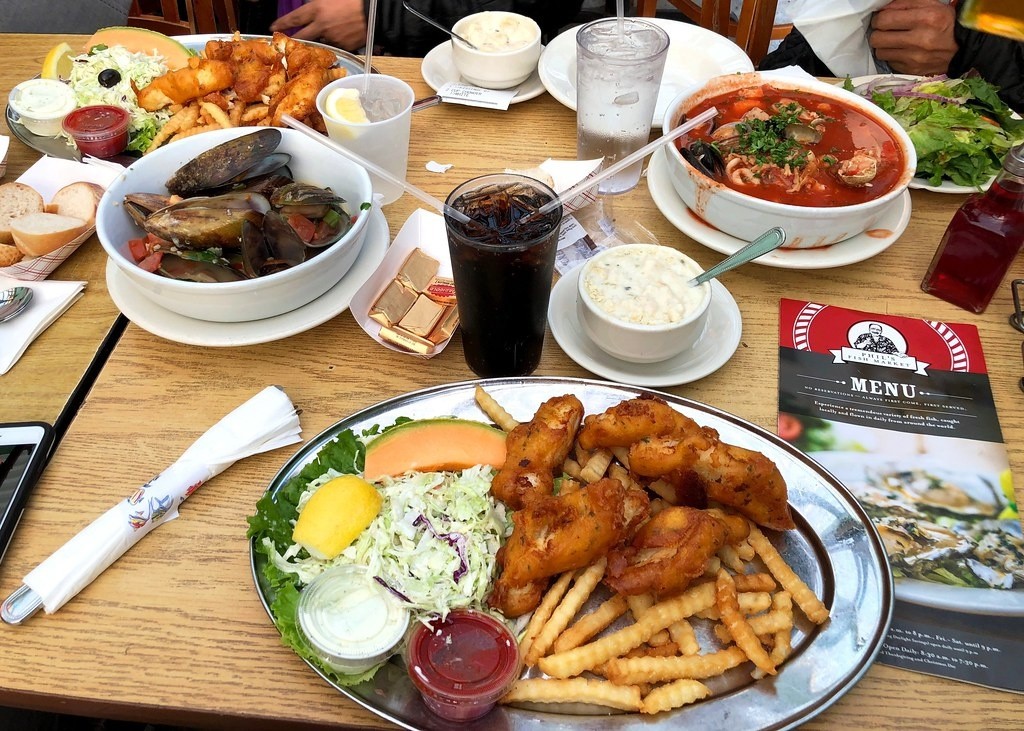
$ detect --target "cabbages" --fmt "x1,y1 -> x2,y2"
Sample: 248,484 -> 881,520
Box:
65,45 -> 173,130
266,466 -> 513,633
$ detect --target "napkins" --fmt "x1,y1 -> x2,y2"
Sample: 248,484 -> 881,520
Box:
23,386 -> 303,615
0,276 -> 89,377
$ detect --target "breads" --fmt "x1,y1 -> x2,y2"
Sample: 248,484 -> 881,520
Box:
0,182 -> 106,267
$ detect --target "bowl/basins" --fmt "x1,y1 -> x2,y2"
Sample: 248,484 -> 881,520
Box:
95,125 -> 373,324
576,242 -> 713,364
405,602 -> 522,723
293,560 -> 412,677
62,105 -> 131,159
662,71 -> 917,249
451,11 -> 541,90
9,78 -> 77,138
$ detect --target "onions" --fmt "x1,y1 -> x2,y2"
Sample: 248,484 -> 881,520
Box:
853,74 -> 959,104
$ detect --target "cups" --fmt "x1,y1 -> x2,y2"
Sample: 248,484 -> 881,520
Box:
576,18 -> 670,196
315,74 -> 416,207
442,173 -> 564,380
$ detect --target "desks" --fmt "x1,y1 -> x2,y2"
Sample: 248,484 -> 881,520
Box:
0,31 -> 122,428
0,51 -> 1024,731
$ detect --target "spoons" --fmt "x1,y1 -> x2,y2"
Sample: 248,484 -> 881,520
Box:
0,287 -> 34,324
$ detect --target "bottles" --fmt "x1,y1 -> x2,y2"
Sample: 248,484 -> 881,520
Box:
920,141 -> 1024,317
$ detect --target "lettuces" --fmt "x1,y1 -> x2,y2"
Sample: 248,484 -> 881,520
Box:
89,43 -> 158,149
875,67 -> 1024,187
244,416 -> 414,687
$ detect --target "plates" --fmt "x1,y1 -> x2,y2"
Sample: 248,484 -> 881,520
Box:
646,145 -> 913,270
536,17 -> 755,129
105,200 -> 389,348
830,73 -> 1022,194
250,377 -> 898,731
5,34 -> 382,168
547,260 -> 743,387
421,40 -> 547,104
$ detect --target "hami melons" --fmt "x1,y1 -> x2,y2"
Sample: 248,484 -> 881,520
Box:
364,419 -> 509,481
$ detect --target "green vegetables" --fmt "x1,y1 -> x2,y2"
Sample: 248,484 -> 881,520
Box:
708,101 -> 839,178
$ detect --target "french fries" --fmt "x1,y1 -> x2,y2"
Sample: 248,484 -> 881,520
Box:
474,384 -> 831,712
143,98 -> 271,155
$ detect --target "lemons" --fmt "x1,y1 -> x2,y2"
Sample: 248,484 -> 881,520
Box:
41,42 -> 74,82
291,474 -> 383,560
325,87 -> 372,127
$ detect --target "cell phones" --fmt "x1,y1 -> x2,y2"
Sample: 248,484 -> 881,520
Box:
0,420 -> 56,563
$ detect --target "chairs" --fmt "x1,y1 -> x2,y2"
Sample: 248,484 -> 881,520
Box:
625,0 -> 794,71
126,0 -> 237,36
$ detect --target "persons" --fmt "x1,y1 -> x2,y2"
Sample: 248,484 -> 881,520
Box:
270,0 -> 583,59
758,0 -> 1024,115
